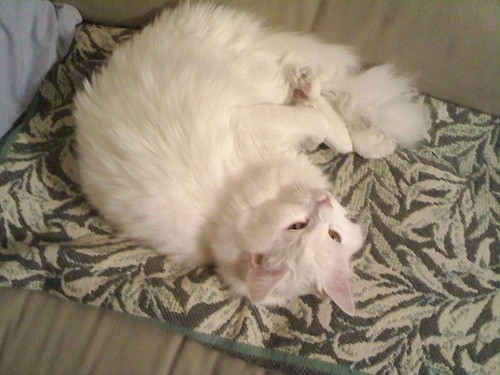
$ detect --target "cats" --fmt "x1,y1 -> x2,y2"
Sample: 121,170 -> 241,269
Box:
70,0 -> 431,317
